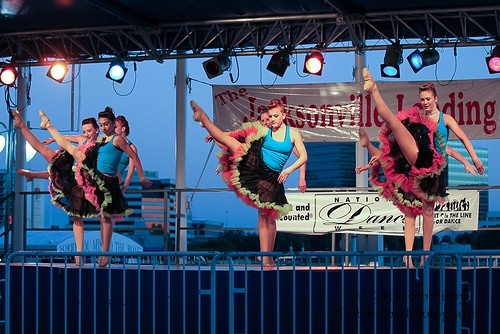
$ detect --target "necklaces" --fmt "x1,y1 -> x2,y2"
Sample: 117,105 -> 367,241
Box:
427,112 -> 438,116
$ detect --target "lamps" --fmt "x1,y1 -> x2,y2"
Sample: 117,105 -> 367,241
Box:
202,54 -> 232,80
303,52 -> 324,76
266,50 -> 291,77
105,58 -> 128,84
0,65 -> 17,87
407,40 -> 440,73
380,41 -> 404,78
485,45 -> 500,74
46,61 -> 69,83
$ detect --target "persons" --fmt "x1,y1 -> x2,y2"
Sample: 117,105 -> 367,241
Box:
12,108 -> 154,267
356,127 -> 417,268
189,98 -> 310,267
362,67 -> 484,267
